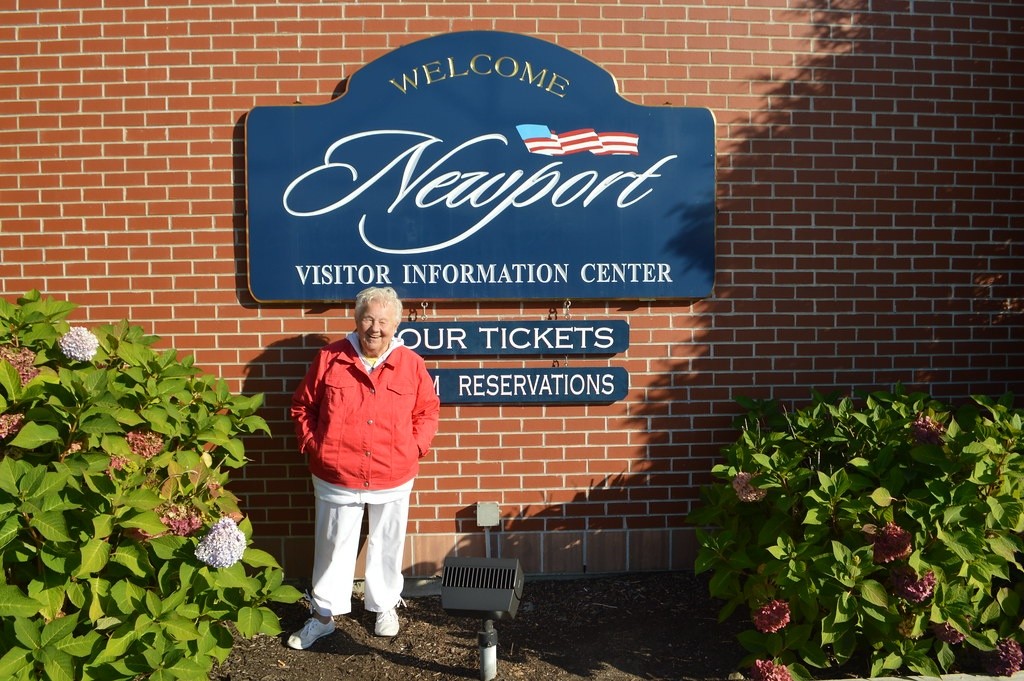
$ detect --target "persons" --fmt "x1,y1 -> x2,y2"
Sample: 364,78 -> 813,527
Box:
287,287 -> 440,649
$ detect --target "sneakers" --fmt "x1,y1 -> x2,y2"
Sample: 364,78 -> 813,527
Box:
375,608 -> 400,637
287,616 -> 335,650
